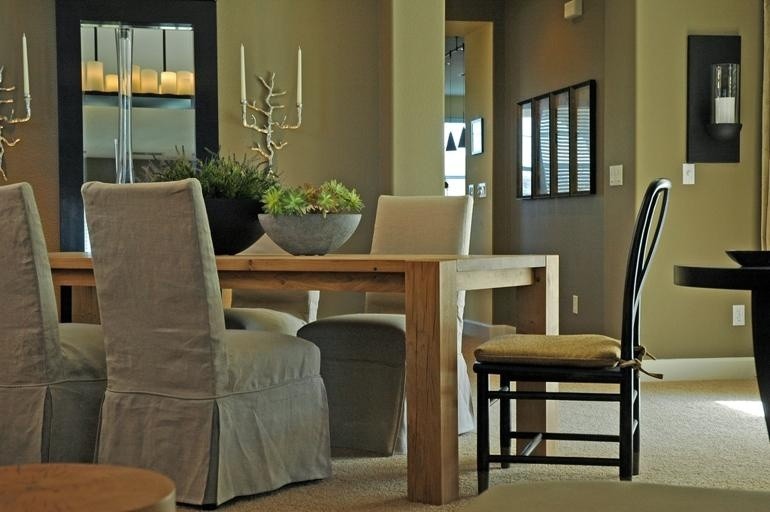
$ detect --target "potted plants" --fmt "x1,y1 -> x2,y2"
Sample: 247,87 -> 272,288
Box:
256,180 -> 364,255
137,152 -> 282,255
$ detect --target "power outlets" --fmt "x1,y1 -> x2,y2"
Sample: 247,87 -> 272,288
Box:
609,164 -> 623,186
572,294 -> 578,314
733,304 -> 745,326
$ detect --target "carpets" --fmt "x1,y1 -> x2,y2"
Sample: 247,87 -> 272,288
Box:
466,480 -> 769,512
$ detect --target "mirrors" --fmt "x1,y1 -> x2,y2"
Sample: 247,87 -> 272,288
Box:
514,79 -> 596,200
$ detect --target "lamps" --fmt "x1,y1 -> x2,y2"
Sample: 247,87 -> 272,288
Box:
457,44 -> 467,149
81,22 -> 196,97
445,51 -> 457,152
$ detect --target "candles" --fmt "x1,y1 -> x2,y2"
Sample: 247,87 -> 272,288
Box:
20,34 -> 29,97
295,45 -> 304,107
240,41 -> 246,101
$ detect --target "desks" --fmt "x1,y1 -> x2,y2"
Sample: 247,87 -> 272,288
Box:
674,265 -> 770,438
46,251 -> 560,506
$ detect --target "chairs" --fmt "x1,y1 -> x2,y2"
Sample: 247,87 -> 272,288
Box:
0,182 -> 110,465
81,180 -> 328,501
299,195 -> 474,453
472,179 -> 672,488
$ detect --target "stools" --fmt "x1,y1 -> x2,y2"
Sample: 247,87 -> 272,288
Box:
0,463 -> 178,511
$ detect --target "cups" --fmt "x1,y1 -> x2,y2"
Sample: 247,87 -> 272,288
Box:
80,61 -> 194,95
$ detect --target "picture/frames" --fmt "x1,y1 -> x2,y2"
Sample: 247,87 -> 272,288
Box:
470,117 -> 484,156
